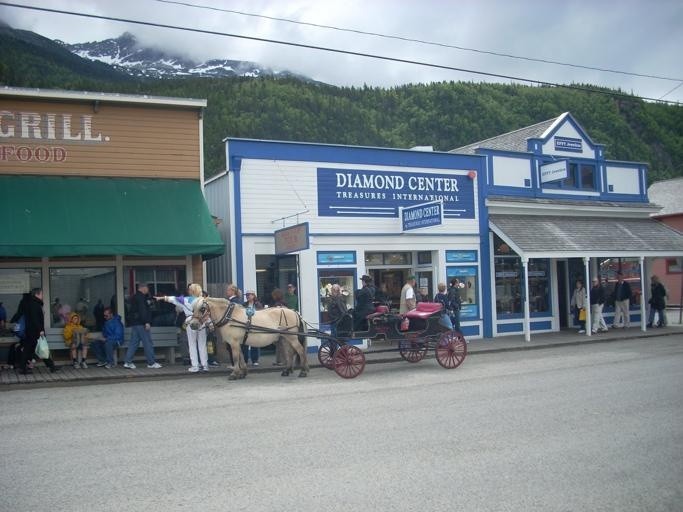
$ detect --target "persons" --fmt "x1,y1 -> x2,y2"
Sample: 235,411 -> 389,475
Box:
2,274 -> 460,374
590,278 -> 608,333
646,275 -> 667,328
571,278 -> 587,334
612,273 -> 633,330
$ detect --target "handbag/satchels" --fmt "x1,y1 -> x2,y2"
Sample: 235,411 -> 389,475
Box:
73,328 -> 87,350
207,333 -> 217,355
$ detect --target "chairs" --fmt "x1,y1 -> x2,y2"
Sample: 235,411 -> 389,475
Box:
364,306 -> 389,320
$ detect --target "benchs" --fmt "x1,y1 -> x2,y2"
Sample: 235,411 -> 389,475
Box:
406,302 -> 443,328
45,327 -> 178,364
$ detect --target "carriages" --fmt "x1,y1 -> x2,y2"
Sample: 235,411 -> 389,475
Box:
189,295 -> 467,378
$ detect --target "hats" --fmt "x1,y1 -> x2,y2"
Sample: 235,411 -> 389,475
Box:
244,289 -> 255,294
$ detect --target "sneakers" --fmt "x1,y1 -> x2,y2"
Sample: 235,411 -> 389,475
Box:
96,361 -> 112,368
20,368 -> 33,374
148,363 -> 162,368
210,361 -> 219,367
51,366 -> 61,373
189,363 -> 209,372
73,361 -> 88,369
123,363 -> 136,369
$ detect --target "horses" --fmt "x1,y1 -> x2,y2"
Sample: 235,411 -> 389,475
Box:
190,292 -> 310,380
